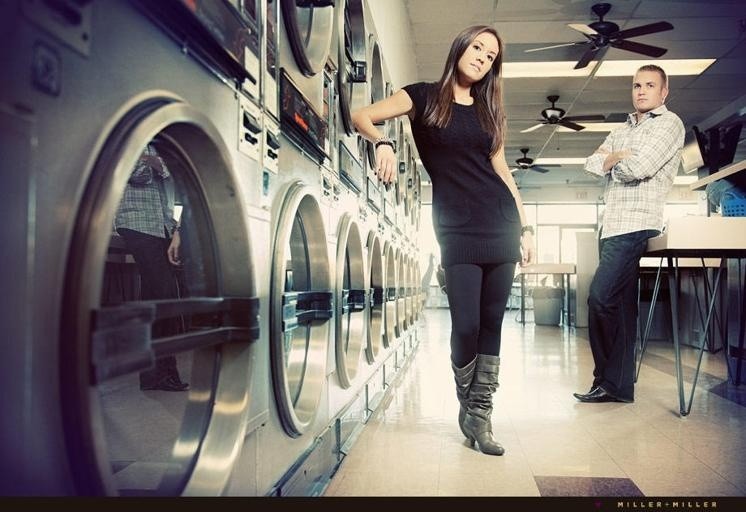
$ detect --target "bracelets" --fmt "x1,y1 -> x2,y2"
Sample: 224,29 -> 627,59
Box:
170,225 -> 183,234
376,136 -> 396,152
521,225 -> 533,235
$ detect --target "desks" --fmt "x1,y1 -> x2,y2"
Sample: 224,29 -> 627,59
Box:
514,261 -> 575,327
635,256 -> 722,354
634,216 -> 744,416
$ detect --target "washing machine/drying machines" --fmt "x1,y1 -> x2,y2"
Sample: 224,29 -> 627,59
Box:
265,0 -> 343,494
0,0 -> 264,512
368,4 -> 421,426
340,1 -> 371,455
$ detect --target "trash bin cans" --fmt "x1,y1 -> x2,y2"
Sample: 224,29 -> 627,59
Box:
532,286 -> 564,325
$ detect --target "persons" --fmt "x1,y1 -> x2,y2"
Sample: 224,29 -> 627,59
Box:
168,208 -> 215,332
349,25 -> 533,454
573,65 -> 685,402
115,143 -> 189,391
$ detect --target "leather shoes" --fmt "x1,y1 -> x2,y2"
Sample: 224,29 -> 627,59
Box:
574,387 -> 625,403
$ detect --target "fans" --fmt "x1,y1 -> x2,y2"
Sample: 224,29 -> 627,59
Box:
519,96 -> 605,133
524,3 -> 675,70
509,149 -> 561,173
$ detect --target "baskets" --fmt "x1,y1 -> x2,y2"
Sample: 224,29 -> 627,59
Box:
721,192 -> 746,217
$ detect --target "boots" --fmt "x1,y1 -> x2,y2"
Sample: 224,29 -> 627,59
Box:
463,354 -> 505,455
450,353 -> 478,440
